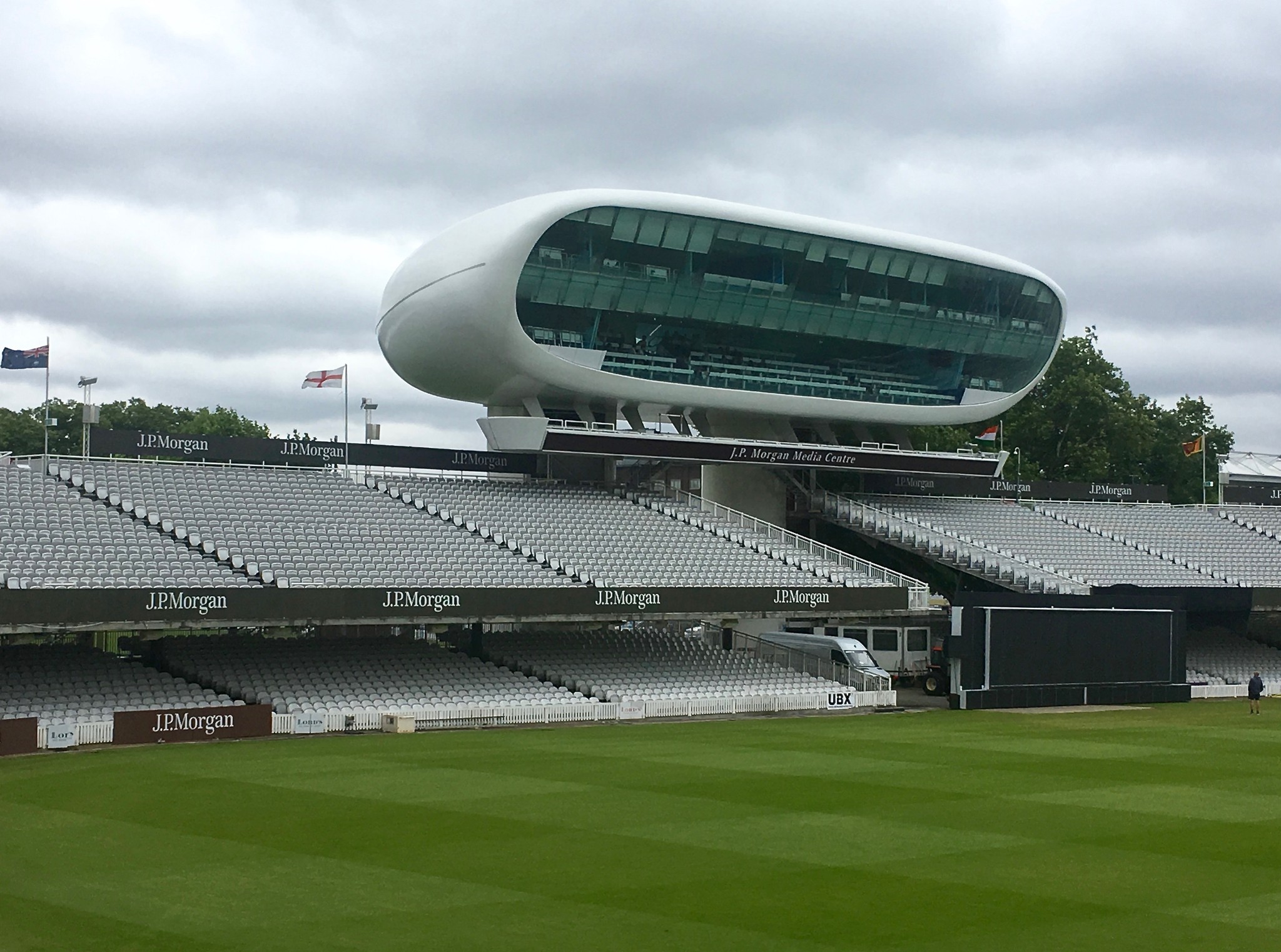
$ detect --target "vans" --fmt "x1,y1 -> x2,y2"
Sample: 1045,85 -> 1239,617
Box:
754,631 -> 890,691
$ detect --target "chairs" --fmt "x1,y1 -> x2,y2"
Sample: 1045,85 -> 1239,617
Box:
1186,639 -> 1281,685
0,628 -> 855,726
811,493 -> 1281,595
0,464 -> 897,589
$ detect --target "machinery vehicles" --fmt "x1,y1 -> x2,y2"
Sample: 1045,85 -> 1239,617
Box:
921,646 -> 951,697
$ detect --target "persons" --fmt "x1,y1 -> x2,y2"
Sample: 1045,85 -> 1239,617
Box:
1248,672 -> 1263,714
597,322 -> 768,384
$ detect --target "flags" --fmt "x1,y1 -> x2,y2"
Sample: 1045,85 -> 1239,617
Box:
0,346 -> 50,369
975,425 -> 998,441
301,366 -> 346,389
1182,436 -> 1203,457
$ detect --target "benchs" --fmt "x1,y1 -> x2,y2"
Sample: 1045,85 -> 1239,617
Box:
596,341 -> 956,407
